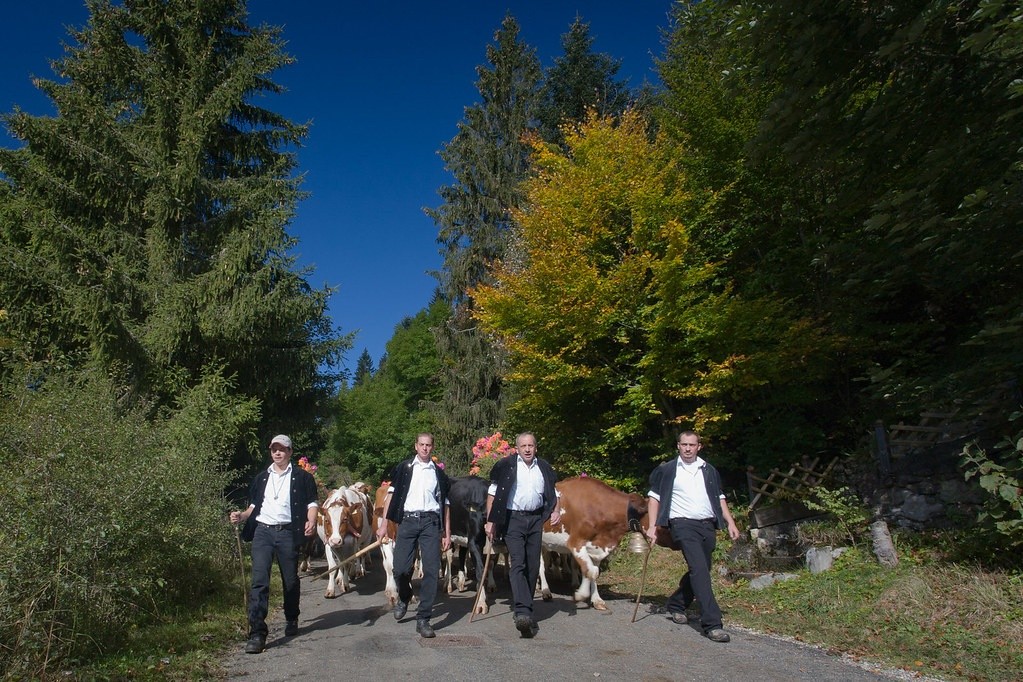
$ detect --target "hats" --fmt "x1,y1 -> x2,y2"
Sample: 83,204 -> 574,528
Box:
268,435 -> 292,449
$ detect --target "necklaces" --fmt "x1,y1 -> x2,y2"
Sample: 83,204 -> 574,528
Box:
272,471 -> 287,499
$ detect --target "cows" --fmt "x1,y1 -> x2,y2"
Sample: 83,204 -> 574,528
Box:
550,551 -> 580,588
486,544 -> 510,593
414,550 -> 424,579
536,476 -> 681,610
439,475 -> 498,614
373,485 -> 417,605
316,482 -> 375,599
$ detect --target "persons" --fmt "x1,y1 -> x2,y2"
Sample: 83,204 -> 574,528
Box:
485,432 -> 561,638
647,432 -> 739,642
376,433 -> 451,638
230,435 -> 318,653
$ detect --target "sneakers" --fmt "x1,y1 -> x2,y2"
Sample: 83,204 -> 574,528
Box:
245,636 -> 264,653
393,599 -> 407,620
416,619 -> 435,638
515,615 -> 535,638
707,628 -> 730,641
285,617 -> 299,637
672,611 -> 688,624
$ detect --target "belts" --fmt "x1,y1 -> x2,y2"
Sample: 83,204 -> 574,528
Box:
258,522 -> 292,531
402,511 -> 436,517
507,507 -> 541,515
